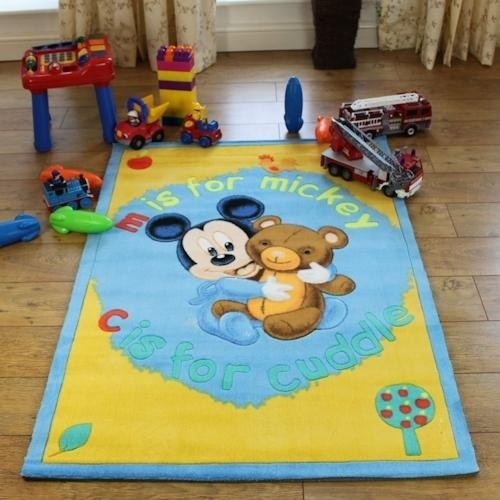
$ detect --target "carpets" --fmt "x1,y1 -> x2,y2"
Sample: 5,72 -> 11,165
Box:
22,139 -> 481,480
